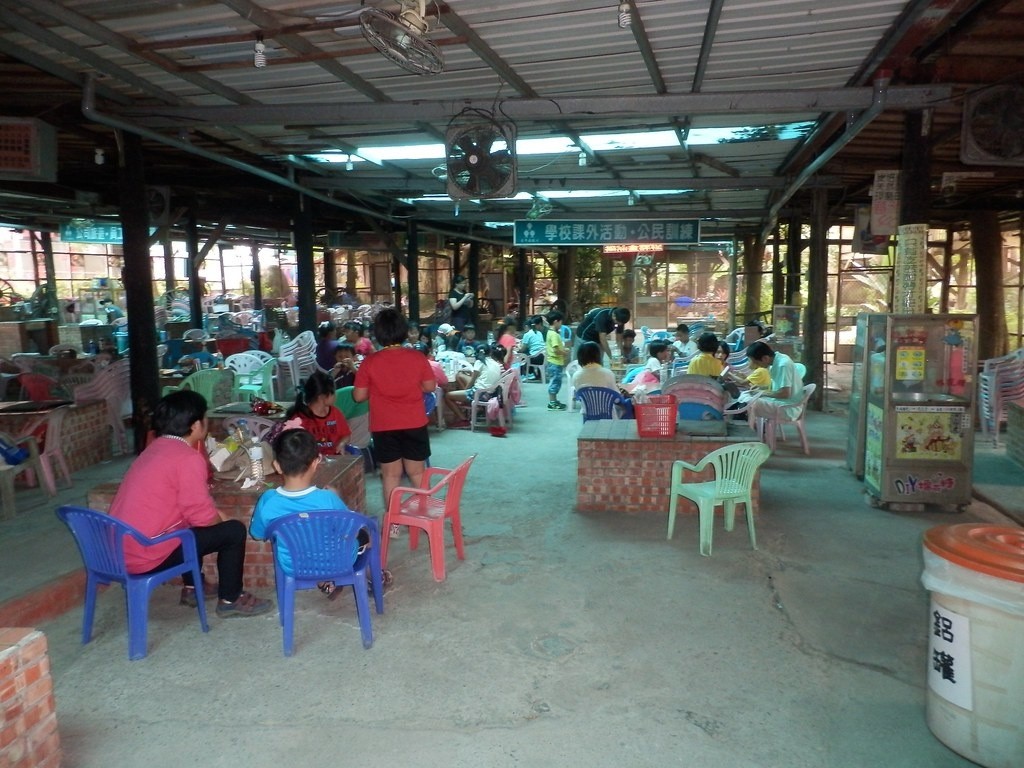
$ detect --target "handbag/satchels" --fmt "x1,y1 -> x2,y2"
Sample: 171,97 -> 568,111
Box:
213,438 -> 277,481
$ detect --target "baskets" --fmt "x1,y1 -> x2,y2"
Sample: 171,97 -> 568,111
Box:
634,394 -> 676,438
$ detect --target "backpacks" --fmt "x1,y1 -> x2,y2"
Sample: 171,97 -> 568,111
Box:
510,367 -> 523,404
0,432 -> 30,466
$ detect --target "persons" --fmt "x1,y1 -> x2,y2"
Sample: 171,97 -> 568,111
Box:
105,391 -> 272,618
315,274 -> 805,432
248,428 -> 393,601
100,298 -> 123,320
353,307 -> 435,540
286,371 -> 352,456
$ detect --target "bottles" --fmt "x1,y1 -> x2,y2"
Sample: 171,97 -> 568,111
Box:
248,437 -> 263,480
88,338 -> 96,354
438,355 -> 459,375
98,338 -> 105,351
236,419 -> 250,441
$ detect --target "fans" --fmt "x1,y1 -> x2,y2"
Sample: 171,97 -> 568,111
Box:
961,85 -> 1024,167
446,122 -> 519,201
146,185 -> 170,227
359,0 -> 445,76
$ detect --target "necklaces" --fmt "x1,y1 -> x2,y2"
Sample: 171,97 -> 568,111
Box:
384,344 -> 401,349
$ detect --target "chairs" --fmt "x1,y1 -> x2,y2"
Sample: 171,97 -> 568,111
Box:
977,347 -> 1024,449
0,304 -> 816,662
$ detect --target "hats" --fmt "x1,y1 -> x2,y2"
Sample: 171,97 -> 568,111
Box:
99,297 -> 114,305
437,324 -> 460,337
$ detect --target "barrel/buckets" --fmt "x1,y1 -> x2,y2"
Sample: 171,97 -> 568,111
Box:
920,523 -> 1024,768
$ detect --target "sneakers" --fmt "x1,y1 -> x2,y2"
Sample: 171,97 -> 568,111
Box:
216,590 -> 274,618
179,573 -> 219,609
547,401 -> 567,411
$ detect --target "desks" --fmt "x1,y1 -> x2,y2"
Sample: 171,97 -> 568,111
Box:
1,399 -> 112,484
0,626 -> 62,768
611,364 -> 640,383
0,319 -> 59,359
619,382 -> 663,394
206,402 -> 297,442
37,355 -> 95,374
165,322 -> 189,339
58,325 -> 114,352
430,377 -> 468,424
86,454 -> 368,588
577,419 -> 761,517
474,320 -> 498,340
159,368 -> 233,408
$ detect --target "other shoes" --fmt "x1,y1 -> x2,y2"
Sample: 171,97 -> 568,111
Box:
521,374 -> 527,382
527,371 -> 535,379
368,568 -> 393,597
318,581 -> 343,602
513,399 -> 528,408
388,523 -> 400,539
447,420 -> 470,429
436,419 -> 446,431
